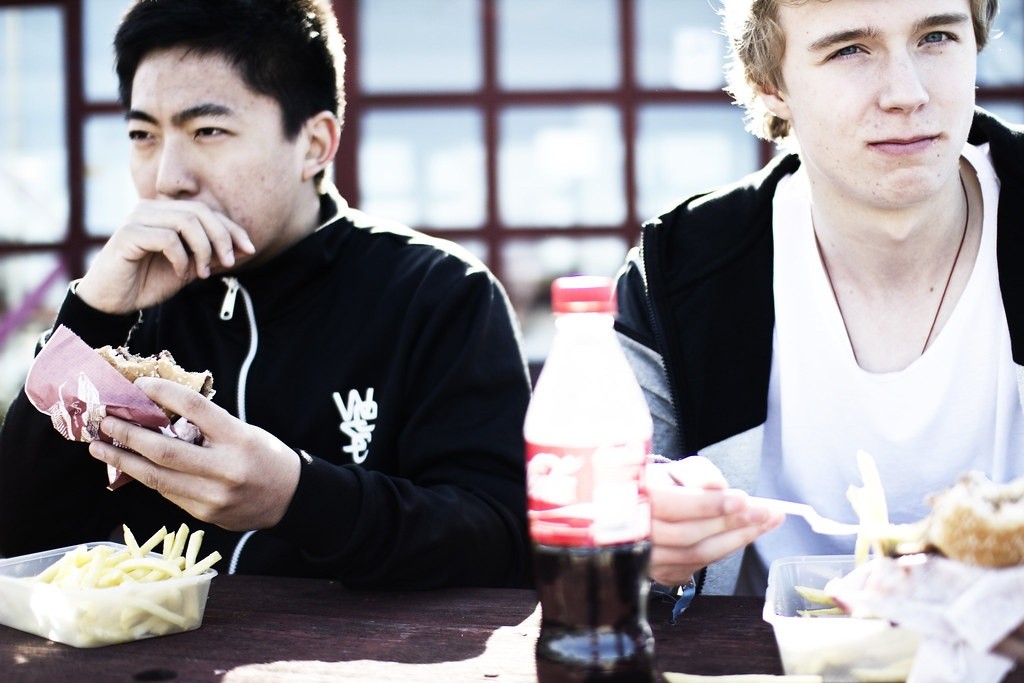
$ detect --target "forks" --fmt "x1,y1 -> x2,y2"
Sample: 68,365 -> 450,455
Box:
665,461 -> 896,538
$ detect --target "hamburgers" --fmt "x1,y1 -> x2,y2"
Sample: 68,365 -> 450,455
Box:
927,469 -> 1024,567
94,346 -> 216,419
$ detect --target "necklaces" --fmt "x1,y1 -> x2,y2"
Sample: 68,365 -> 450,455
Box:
920,170 -> 970,356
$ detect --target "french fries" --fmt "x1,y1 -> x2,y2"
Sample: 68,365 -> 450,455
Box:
0,522 -> 221,647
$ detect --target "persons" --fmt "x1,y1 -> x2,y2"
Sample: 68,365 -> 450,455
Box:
608,0 -> 1023,596
2,2 -> 543,601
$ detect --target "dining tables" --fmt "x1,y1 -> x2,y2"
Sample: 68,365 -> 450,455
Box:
0,577 -> 782,683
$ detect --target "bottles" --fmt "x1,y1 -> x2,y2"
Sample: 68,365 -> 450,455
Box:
524,275 -> 670,683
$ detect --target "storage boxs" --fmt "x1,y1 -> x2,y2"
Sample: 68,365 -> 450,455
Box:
0,542 -> 218,648
763,554 -> 914,682
